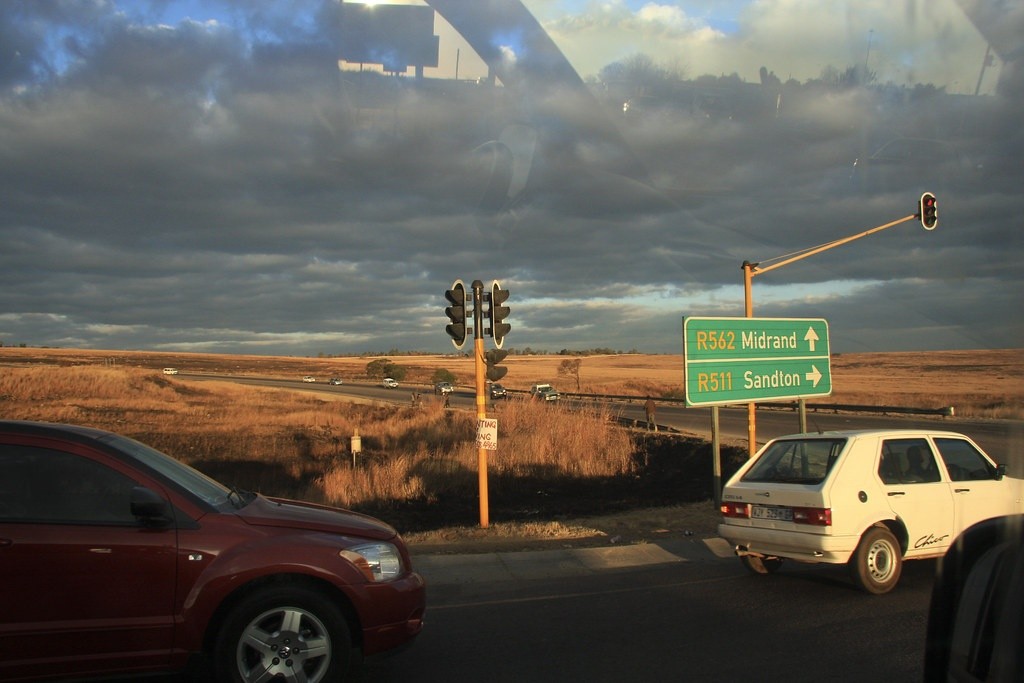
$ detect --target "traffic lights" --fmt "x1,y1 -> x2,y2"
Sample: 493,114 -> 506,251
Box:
487,349 -> 508,382
921,192 -> 937,230
492,280 -> 511,349
445,279 -> 467,350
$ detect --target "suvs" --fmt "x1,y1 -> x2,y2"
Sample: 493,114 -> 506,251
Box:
717,395 -> 1024,594
435,382 -> 453,395
384,379 -> 398,388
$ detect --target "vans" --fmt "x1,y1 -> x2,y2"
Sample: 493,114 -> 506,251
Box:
163,368 -> 179,375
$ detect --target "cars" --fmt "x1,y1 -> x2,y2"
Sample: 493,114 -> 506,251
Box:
0,420 -> 427,683
303,376 -> 315,383
330,379 -> 342,385
490,384 -> 507,399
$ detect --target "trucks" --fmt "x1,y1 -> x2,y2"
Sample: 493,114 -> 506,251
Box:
531,384 -> 560,402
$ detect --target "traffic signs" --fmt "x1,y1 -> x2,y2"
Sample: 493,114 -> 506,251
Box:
683,316 -> 830,408
